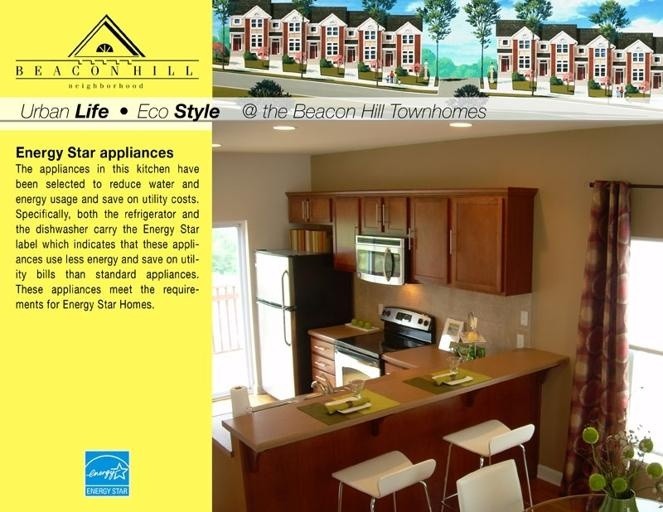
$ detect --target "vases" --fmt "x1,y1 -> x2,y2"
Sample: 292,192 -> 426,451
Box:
598,488 -> 639,512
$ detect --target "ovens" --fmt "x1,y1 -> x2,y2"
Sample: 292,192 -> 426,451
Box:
335,349 -> 383,391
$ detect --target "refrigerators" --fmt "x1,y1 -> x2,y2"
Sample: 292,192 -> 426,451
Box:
255,249 -> 336,403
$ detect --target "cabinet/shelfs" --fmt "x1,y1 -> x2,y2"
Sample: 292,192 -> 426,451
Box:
382,342 -> 459,376
286,186 -> 537,297
308,322 -> 382,391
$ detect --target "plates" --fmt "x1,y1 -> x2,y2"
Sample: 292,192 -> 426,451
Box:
324,396 -> 373,415
433,372 -> 473,387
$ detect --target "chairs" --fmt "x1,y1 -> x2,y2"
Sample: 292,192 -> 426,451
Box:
456,459 -> 525,512
440,418 -> 535,512
332,451 -> 437,512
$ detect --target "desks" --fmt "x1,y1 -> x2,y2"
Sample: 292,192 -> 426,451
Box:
524,493 -> 663,512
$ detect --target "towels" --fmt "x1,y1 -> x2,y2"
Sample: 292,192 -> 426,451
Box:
327,397 -> 369,415
434,372 -> 466,385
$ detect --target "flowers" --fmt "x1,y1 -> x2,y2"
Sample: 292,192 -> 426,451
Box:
449,311 -> 488,362
582,424 -> 663,509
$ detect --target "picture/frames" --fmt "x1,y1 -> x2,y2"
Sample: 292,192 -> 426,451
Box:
437,317 -> 465,354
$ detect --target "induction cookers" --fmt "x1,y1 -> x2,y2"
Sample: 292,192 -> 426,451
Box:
337,329 -> 432,355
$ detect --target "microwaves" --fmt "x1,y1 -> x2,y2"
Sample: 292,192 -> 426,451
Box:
356,235 -> 409,287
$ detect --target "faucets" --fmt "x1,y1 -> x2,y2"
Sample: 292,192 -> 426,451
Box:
310,373 -> 335,394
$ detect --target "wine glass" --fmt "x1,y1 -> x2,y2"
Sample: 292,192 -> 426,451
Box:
446,356 -> 464,376
350,379 -> 365,396
